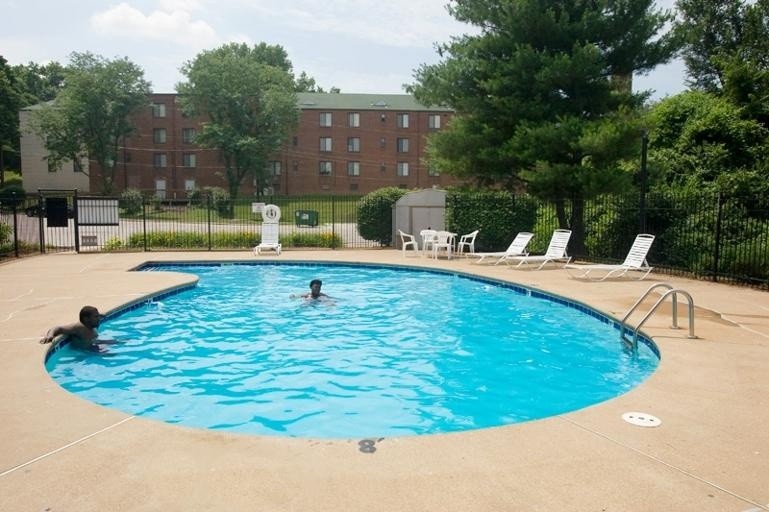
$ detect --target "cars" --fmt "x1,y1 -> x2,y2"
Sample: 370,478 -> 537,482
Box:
24,201 -> 75,218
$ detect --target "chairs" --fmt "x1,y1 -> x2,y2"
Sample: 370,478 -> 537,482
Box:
398,230 -> 479,260
465,231 -> 535,268
564,233 -> 655,282
506,229 -> 572,271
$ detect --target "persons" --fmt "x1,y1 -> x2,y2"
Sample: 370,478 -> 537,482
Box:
39,305 -> 116,357
290,279 -> 338,302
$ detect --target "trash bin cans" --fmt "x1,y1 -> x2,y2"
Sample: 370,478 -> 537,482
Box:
295,210 -> 319,228
